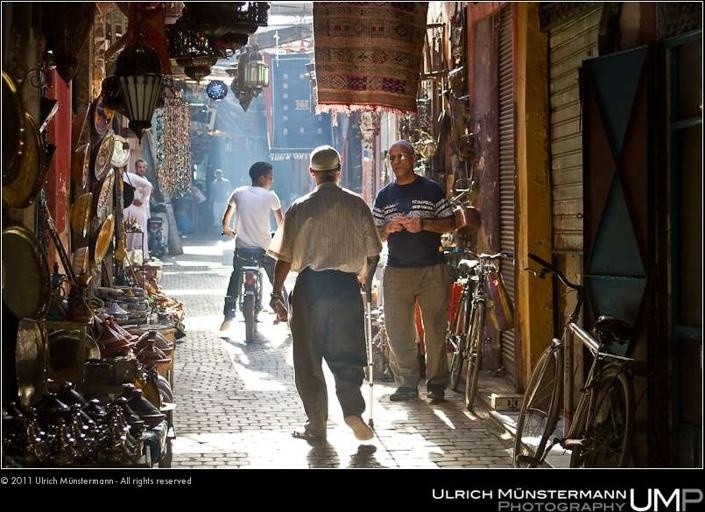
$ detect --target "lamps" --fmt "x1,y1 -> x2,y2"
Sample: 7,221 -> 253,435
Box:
115,46 -> 164,146
230,43 -> 268,112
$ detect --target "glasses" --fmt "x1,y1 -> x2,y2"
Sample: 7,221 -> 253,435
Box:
391,153 -> 409,160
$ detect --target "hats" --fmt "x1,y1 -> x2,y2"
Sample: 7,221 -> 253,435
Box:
310,146 -> 340,172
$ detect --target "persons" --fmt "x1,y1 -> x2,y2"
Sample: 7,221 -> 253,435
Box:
173,168 -> 234,240
134,160 -> 154,251
218,162 -> 289,331
122,163 -> 152,258
371,138 -> 458,401
265,144 -> 384,443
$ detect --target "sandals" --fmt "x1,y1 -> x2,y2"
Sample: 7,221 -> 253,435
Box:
292,429 -> 327,441
345,414 -> 373,441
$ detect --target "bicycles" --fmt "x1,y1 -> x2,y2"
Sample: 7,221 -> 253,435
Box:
439,240 -> 515,411
514,253 -> 653,469
221,228 -> 276,344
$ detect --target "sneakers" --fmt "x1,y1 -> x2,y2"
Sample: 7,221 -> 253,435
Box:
219,318 -> 232,332
389,388 -> 419,401
427,388 -> 444,399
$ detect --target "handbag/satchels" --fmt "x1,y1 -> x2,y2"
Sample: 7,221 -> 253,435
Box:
486,272 -> 514,331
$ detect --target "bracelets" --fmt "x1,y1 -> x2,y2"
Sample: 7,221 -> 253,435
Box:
270,291 -> 283,298
